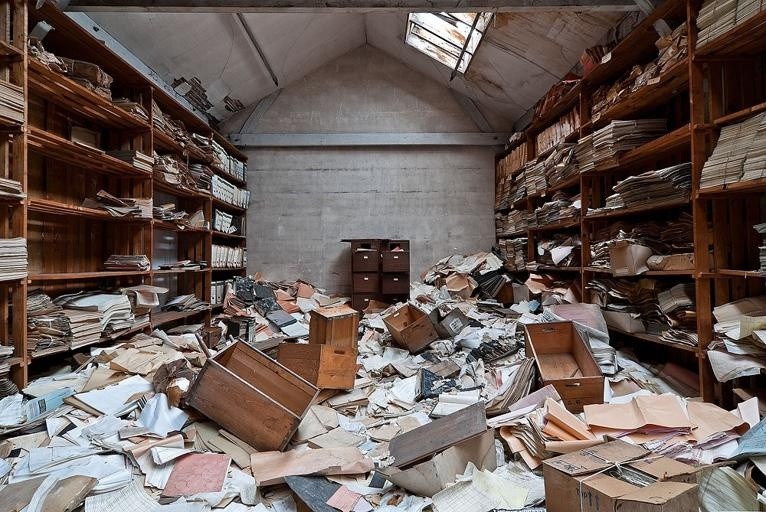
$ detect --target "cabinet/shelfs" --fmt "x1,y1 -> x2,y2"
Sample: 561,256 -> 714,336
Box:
341,236 -> 410,312
174,304 -> 360,450
381,302 -> 470,355
492,1 -> 766,436
0,1 -> 251,399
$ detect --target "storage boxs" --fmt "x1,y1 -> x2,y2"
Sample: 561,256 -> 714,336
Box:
518,316 -> 696,512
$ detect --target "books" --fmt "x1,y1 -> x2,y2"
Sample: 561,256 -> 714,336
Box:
1,1 -> 251,279
1,253 -> 766,512
493,1 -> 765,274
